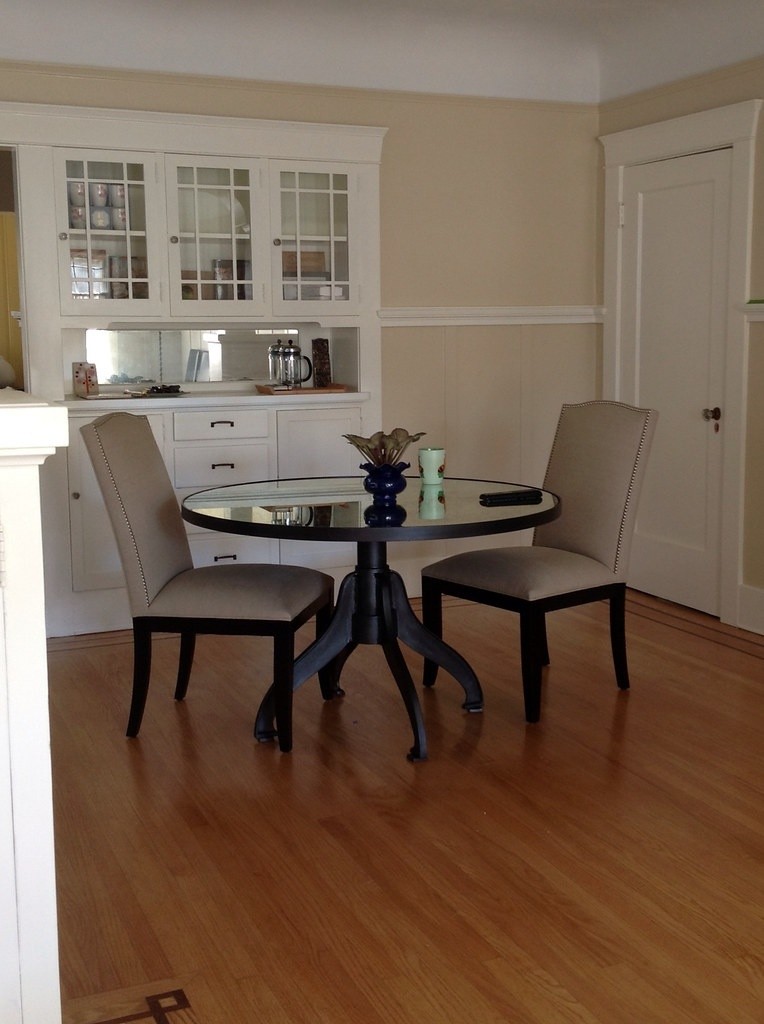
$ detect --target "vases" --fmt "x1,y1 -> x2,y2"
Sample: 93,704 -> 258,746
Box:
363,500 -> 407,527
359,461 -> 411,499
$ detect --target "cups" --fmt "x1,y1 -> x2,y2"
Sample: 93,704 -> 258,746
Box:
111,208 -> 126,230
69,182 -> 85,206
108,185 -> 126,207
70,207 -> 85,228
419,485 -> 446,520
418,447 -> 445,485
89,184 -> 108,206
91,207 -> 111,229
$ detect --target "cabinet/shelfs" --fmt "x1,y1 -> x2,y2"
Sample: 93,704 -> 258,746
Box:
166,403 -> 278,574
52,144 -> 165,324
270,158 -> 359,328
165,154 -> 269,324
278,399 -> 363,568
42,403 -> 171,639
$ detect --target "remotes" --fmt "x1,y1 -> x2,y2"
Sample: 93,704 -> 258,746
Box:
480,488 -> 542,504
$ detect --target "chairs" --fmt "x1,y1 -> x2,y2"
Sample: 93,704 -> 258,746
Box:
421,398 -> 659,722
78,412 -> 346,744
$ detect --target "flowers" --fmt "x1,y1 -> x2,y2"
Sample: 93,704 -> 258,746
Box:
341,428 -> 427,464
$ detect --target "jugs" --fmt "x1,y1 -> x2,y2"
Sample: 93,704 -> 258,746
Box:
277,340 -> 313,387
267,339 -> 282,379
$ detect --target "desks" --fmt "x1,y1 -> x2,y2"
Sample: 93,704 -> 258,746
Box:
180,475 -> 561,759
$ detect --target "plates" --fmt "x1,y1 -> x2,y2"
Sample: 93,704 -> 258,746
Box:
142,390 -> 191,397
